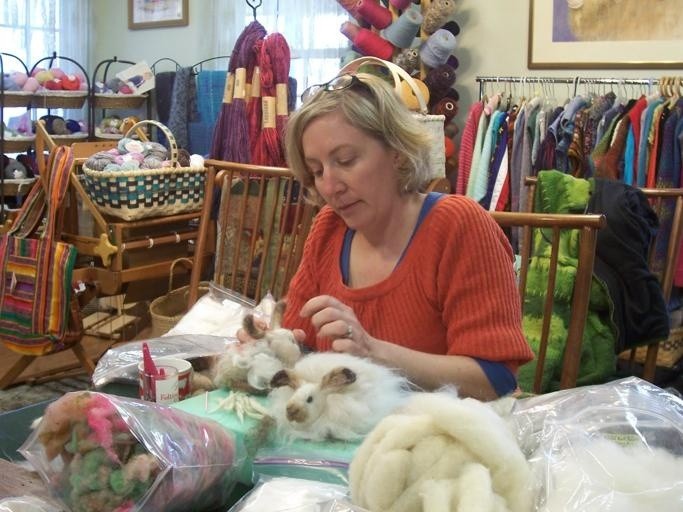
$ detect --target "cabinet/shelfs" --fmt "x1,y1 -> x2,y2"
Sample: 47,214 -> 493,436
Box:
35,116 -> 217,296
1,51 -> 151,209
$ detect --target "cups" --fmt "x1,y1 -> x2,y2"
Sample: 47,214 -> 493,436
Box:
138,356 -> 193,402
143,366 -> 179,404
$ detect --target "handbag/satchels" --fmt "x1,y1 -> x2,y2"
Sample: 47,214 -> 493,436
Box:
1,236 -> 83,357
339,55 -> 446,180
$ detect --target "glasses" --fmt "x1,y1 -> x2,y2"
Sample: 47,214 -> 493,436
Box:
301,74 -> 369,103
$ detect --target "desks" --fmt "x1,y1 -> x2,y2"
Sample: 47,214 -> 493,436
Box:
0,380 -> 252,512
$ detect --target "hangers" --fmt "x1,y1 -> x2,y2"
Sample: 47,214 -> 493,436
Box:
480,75 -> 683,113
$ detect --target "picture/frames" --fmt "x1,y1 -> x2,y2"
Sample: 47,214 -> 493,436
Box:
527,0 -> 681,66
128,0 -> 190,28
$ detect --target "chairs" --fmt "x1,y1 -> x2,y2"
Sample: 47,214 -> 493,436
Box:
187,157 -> 316,312
480,210 -> 606,395
518,181 -> 683,394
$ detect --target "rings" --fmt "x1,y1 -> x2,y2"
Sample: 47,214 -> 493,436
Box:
346,326 -> 353,339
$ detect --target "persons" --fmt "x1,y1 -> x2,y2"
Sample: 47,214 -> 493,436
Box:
237,71 -> 534,402
